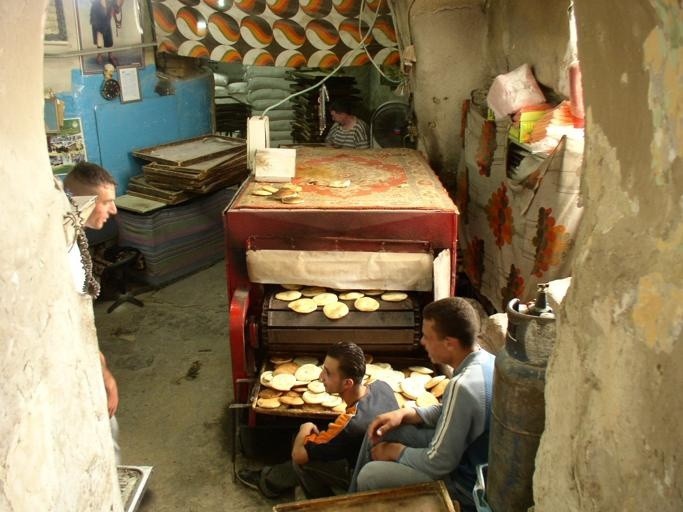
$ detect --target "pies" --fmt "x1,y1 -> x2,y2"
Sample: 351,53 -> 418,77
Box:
257,355 -> 451,413
274,284 -> 408,321
253,176 -> 350,204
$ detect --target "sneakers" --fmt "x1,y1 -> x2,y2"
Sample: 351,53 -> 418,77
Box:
236,466 -> 264,490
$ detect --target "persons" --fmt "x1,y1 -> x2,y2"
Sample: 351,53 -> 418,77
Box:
237,337 -> 398,497
356,295 -> 500,512
62,161 -> 120,468
325,97 -> 370,150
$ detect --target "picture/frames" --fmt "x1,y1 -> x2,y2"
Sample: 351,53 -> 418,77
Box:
73,0 -> 145,75
116,65 -> 142,103
46,117 -> 88,175
45,0 -> 67,41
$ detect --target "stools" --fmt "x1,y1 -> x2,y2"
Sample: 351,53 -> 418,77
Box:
92,245 -> 151,313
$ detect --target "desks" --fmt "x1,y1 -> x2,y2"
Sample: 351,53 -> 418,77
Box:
114,186 -> 238,287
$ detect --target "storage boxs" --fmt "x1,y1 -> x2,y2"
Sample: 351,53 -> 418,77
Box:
509,112 -> 545,142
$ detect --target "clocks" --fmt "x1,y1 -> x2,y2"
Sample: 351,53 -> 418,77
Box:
101,79 -> 120,100
